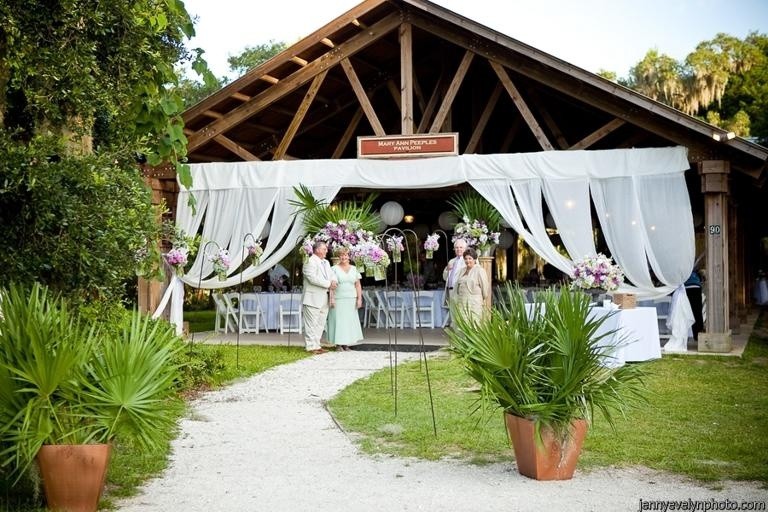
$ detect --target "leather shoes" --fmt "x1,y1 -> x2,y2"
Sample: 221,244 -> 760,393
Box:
312,345 -> 351,353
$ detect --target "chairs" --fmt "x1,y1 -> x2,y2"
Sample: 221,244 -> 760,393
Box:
655,296 -> 672,340
211,292 -> 269,334
361,290 -> 451,331
277,293 -> 304,336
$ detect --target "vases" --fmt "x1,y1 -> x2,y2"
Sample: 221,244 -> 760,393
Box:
426,250 -> 433,259
219,271 -> 227,282
252,258 -> 260,266
176,266 -> 184,278
584,287 -> 607,306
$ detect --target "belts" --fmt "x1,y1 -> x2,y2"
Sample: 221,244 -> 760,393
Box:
448,287 -> 453,289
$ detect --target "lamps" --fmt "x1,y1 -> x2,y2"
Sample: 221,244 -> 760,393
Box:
380,201 -> 404,226
495,205 -> 522,250
712,131 -> 735,142
439,211 -> 457,230
373,212 -> 387,233
257,219 -> 270,240
547,213 -> 557,230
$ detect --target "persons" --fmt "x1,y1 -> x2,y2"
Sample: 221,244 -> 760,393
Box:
302,239 -> 338,354
328,247 -> 364,352
444,237 -> 480,306
419,251 -> 442,285
451,247 -> 492,334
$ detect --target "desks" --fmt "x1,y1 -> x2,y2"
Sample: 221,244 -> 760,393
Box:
238,292 -> 304,332
514,302 -> 661,369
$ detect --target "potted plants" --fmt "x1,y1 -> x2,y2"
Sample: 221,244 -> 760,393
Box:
0,277 -> 194,512
287,183 -> 386,265
447,188 -> 505,256
440,277 -> 659,480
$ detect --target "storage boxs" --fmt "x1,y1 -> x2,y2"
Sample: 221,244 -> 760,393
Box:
613,293 -> 637,309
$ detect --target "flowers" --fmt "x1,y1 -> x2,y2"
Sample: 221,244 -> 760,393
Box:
569,252 -> 625,291
299,220 -> 424,289
208,247 -> 229,281
450,214 -> 501,257
425,233 -> 440,259
246,241 -> 263,265
167,247 -> 188,276
